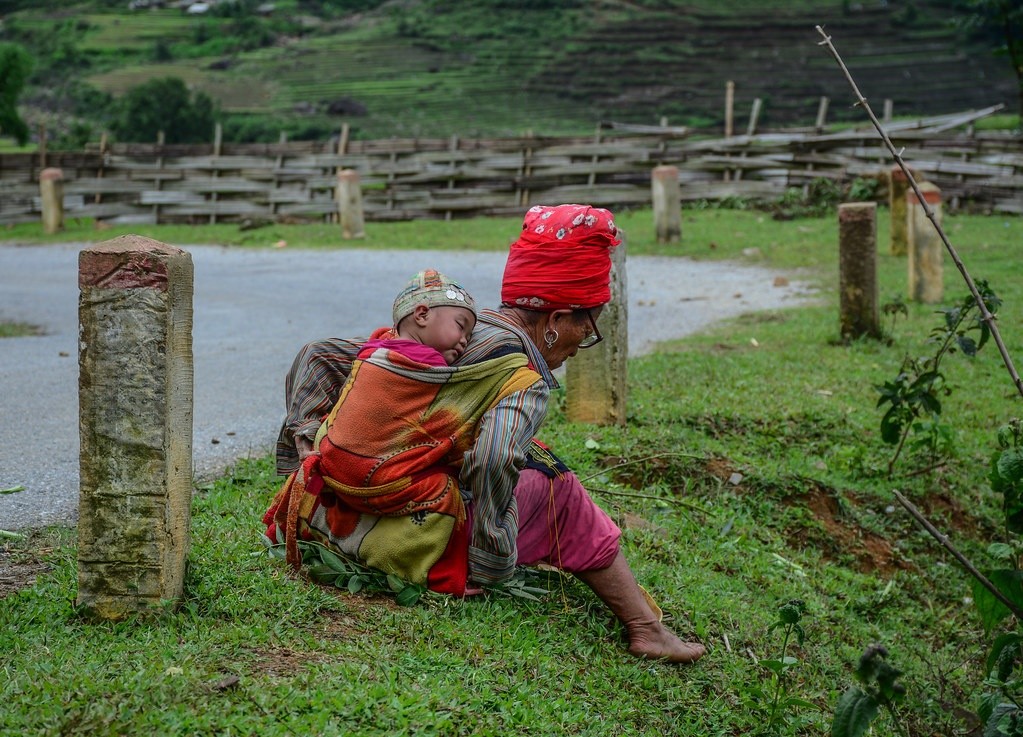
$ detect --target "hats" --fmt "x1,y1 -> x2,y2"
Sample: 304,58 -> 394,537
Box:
391,271 -> 481,327
501,202 -> 617,312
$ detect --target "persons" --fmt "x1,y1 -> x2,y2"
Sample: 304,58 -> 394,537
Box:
274,203 -> 707,665
307,267 -> 483,598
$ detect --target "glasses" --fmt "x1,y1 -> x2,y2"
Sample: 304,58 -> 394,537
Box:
576,306 -> 603,349
